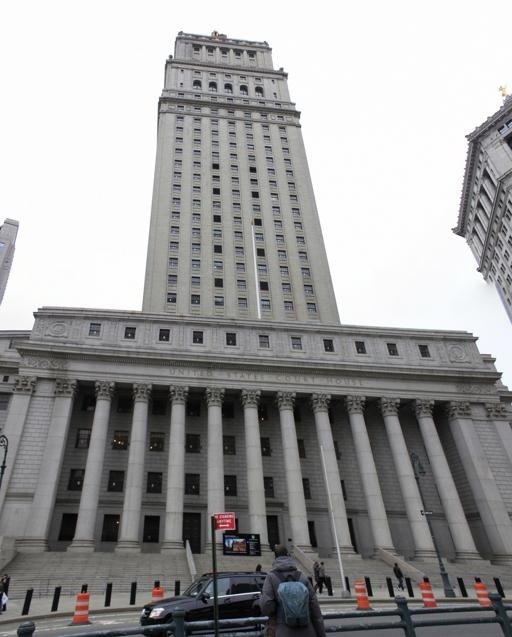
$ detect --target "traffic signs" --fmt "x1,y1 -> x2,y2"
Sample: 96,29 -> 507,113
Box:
214,512 -> 236,531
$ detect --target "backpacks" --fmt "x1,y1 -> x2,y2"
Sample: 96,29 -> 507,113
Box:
270,569 -> 310,627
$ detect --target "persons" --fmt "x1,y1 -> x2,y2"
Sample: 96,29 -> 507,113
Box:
318,561 -> 326,593
0,572 -> 10,615
313,561 -> 320,591
256,563 -> 261,571
393,562 -> 404,591
260,543 -> 326,636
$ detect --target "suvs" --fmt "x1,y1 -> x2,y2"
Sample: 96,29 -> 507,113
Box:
141,571 -> 268,637
